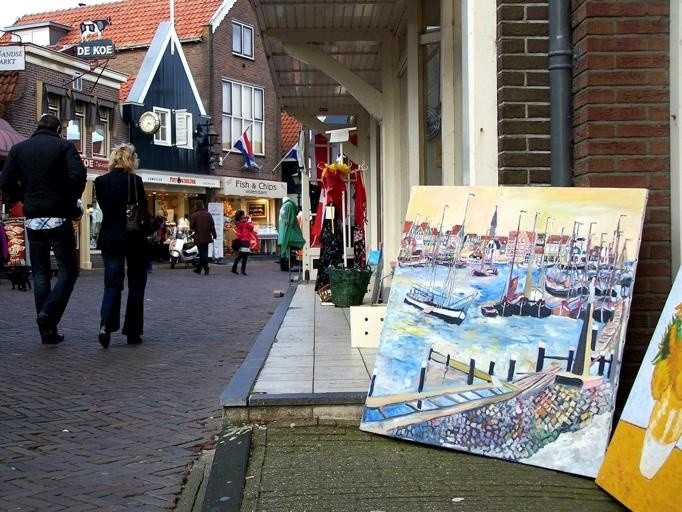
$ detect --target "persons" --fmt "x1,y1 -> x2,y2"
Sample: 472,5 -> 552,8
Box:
189,199 -> 218,276
230,209 -> 254,276
1,114 -> 88,345
277,197 -> 307,258
93,142 -> 156,348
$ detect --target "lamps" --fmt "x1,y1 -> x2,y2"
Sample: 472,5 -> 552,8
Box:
177,177 -> 181,183
194,119 -> 219,146
292,166 -> 304,185
315,108 -> 328,124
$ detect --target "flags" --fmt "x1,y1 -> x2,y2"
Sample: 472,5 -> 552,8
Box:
286,143 -> 299,161
233,132 -> 253,168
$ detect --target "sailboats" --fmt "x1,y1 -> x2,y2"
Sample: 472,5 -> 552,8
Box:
400,193 -> 633,327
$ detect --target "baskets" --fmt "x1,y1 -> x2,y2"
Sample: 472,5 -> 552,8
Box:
326,263 -> 373,306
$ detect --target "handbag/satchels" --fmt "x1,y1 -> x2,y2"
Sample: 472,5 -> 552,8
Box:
231,238 -> 242,251
126,203 -> 147,231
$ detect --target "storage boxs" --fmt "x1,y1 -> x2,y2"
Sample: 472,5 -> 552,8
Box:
349,302 -> 386,349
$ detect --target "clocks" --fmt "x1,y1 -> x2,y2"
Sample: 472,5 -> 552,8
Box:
135,110 -> 161,137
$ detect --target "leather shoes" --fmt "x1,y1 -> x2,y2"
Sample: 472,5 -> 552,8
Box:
37,313 -> 65,345
99,327 -> 143,349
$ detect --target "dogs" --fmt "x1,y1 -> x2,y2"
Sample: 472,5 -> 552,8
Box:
5,268 -> 34,291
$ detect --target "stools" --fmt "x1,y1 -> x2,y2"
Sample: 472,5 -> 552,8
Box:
8,265 -> 60,293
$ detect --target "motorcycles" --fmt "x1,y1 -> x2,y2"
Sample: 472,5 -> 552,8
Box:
167,226 -> 200,269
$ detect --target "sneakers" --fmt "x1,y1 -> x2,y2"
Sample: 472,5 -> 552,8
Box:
231,270 -> 248,276
193,269 -> 209,275
